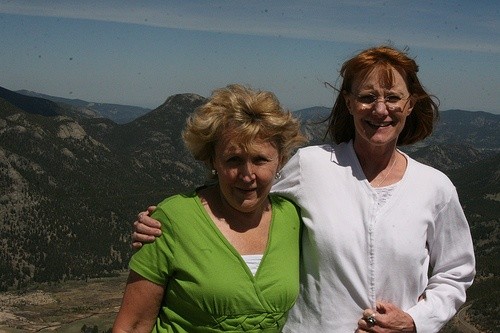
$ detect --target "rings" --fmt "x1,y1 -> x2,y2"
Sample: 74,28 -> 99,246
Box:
367,310 -> 378,326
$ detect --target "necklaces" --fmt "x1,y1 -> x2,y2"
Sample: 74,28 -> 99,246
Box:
377,151 -> 398,186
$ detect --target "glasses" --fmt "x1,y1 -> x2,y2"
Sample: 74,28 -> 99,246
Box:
348,90 -> 410,108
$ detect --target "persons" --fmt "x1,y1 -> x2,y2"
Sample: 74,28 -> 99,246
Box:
111,84 -> 303,332
131,45 -> 476,333
